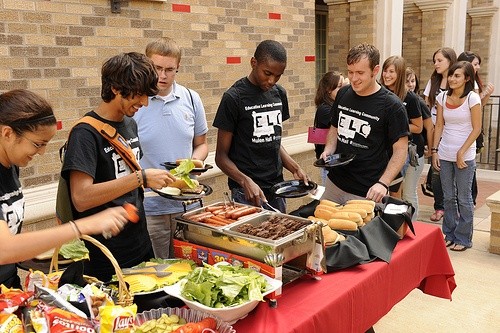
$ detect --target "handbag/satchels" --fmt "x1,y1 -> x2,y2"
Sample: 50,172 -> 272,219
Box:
308,102 -> 332,145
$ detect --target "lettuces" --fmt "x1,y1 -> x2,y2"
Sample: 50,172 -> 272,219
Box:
180,261 -> 269,308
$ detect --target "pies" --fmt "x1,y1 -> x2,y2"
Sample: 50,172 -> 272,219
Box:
111,261 -> 191,294
308,199 -> 375,246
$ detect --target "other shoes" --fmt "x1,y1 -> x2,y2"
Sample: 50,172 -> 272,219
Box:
445,240 -> 467,252
430,209 -> 444,221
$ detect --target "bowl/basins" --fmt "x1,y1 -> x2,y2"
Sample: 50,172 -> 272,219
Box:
125,307 -> 236,333
109,270 -> 187,319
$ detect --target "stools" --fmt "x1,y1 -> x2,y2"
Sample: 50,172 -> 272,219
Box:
485,190 -> 500,256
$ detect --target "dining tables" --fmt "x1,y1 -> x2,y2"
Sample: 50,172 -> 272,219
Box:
135,220 -> 441,333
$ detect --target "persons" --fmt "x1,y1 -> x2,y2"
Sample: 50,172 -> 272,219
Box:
311,69 -> 351,190
211,39 -> 318,216
129,36 -> 212,261
61,52 -> 178,280
455,50 -> 495,209
378,53 -> 423,200
403,67 -> 434,224
419,48 -> 461,224
432,57 -> 484,250
0,86 -> 130,296
317,44 -> 414,210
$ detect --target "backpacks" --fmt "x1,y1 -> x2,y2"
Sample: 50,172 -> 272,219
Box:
55,115 -> 145,225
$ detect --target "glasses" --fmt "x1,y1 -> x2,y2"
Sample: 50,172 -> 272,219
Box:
10,126 -> 47,149
155,66 -> 176,76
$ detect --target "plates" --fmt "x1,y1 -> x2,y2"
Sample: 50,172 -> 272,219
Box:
314,152 -> 358,168
149,180 -> 214,200
159,160 -> 213,173
271,179 -> 318,198
163,260 -> 284,325
12,248 -> 81,274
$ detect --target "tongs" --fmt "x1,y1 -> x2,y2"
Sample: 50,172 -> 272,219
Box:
257,196 -> 281,215
120,263 -> 174,277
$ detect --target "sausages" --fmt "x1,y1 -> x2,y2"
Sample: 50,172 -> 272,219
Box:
186,204 -> 261,226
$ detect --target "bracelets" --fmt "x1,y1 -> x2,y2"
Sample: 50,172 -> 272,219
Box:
431,147 -> 439,154
135,169 -> 142,189
68,219 -> 84,241
139,168 -> 148,189
377,180 -> 390,191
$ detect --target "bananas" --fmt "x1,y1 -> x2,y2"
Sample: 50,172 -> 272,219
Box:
139,313 -> 181,333
